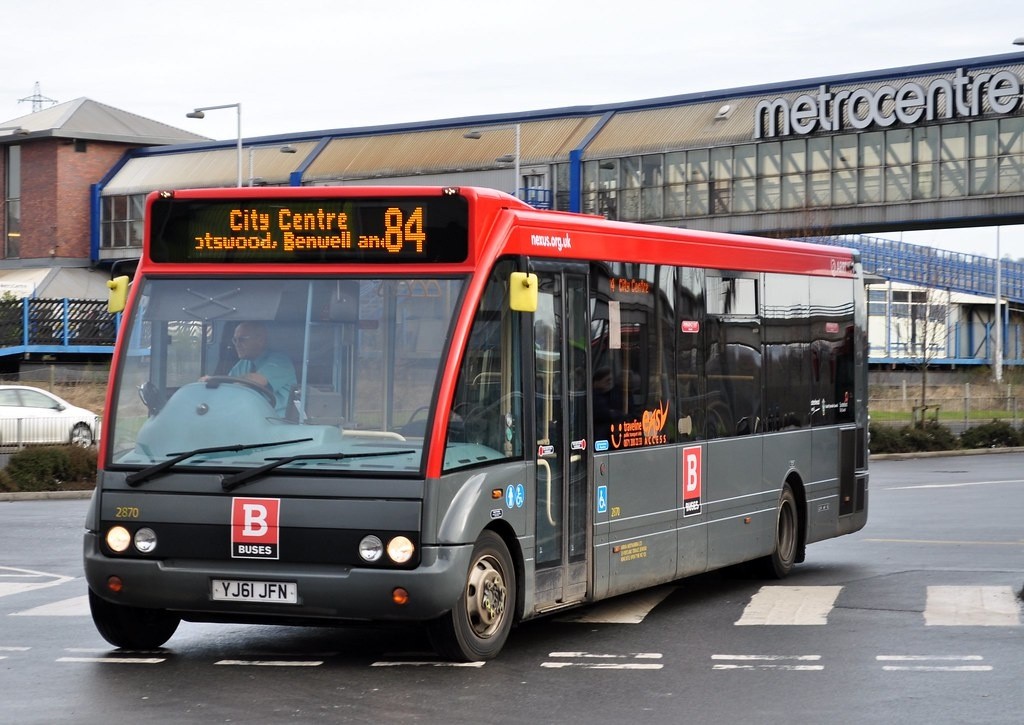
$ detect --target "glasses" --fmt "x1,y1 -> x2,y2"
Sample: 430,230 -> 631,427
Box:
231,334 -> 257,345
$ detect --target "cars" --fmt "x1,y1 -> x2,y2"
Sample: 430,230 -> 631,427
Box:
0,385 -> 102,451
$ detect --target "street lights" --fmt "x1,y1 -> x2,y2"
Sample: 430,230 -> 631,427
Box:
463,125 -> 521,202
186,103 -> 241,189
247,145 -> 296,187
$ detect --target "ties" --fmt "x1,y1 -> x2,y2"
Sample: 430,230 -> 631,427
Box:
249,362 -> 254,374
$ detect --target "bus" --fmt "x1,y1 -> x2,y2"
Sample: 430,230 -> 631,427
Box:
84,187 -> 871,666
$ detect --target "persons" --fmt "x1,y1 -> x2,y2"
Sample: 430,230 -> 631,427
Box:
199,321 -> 298,417
536,367 -> 635,424
